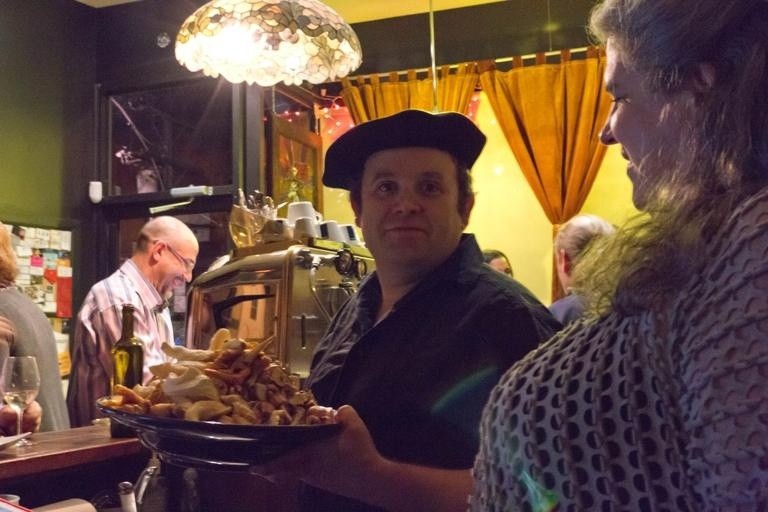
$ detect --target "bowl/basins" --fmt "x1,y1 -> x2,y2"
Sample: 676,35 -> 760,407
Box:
96,393 -> 342,470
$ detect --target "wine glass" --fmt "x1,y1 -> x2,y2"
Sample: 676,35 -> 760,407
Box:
0,340 -> 40,447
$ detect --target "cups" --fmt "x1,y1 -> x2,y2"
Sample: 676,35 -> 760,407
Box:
263,201 -> 366,244
1,494 -> 20,505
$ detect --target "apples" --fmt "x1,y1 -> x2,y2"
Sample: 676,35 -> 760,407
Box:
1,401 -> 44,437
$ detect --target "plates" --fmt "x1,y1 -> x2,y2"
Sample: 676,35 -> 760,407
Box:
0,432 -> 32,453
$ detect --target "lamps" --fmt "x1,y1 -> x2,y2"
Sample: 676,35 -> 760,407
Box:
172,1 -> 364,91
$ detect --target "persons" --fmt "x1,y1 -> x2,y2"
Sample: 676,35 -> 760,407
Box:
248,110 -> 565,512
65,215 -> 200,427
0,316 -> 42,437
0,221 -> 71,432
481,251 -> 513,279
546,213 -> 620,327
467,0 -> 768,512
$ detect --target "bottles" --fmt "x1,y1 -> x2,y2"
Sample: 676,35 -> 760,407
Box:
109,305 -> 144,436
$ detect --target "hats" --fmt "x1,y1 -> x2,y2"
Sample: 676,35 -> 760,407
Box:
320,110 -> 487,191
